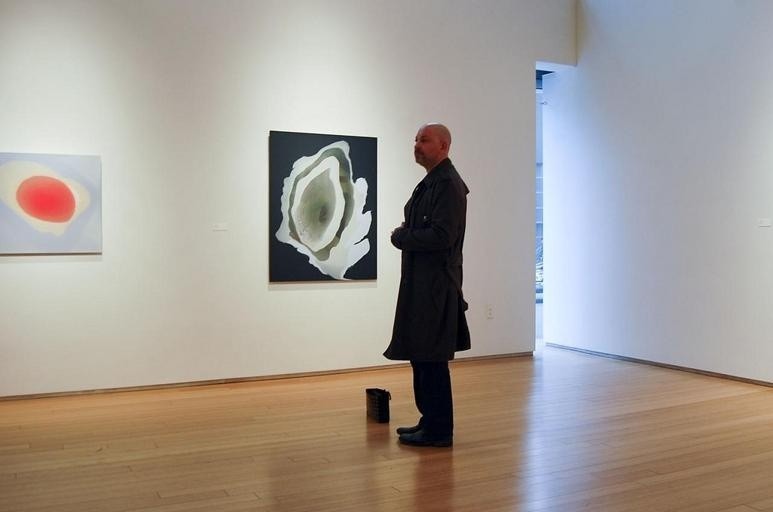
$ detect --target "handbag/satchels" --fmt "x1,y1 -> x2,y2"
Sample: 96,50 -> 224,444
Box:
365,388 -> 390,423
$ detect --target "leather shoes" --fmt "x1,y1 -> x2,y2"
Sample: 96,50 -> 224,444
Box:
396,422 -> 452,447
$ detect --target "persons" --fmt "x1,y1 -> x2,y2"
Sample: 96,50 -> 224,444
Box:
383,122 -> 471,448
